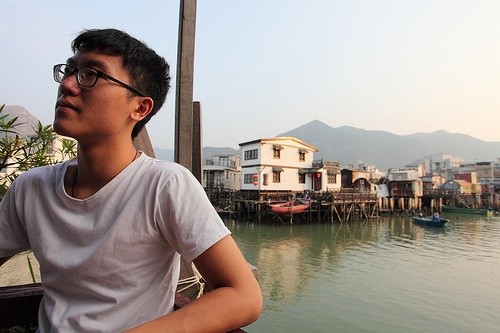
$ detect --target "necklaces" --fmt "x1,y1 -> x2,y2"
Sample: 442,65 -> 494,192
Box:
72,151 -> 140,199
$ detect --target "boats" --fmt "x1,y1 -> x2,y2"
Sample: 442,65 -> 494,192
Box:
272,200 -> 310,214
412,215 -> 449,227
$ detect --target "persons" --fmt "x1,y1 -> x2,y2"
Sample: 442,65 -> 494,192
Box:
0,30 -> 264,333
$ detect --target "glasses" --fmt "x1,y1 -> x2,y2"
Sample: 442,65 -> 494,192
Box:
53,64 -> 145,103
